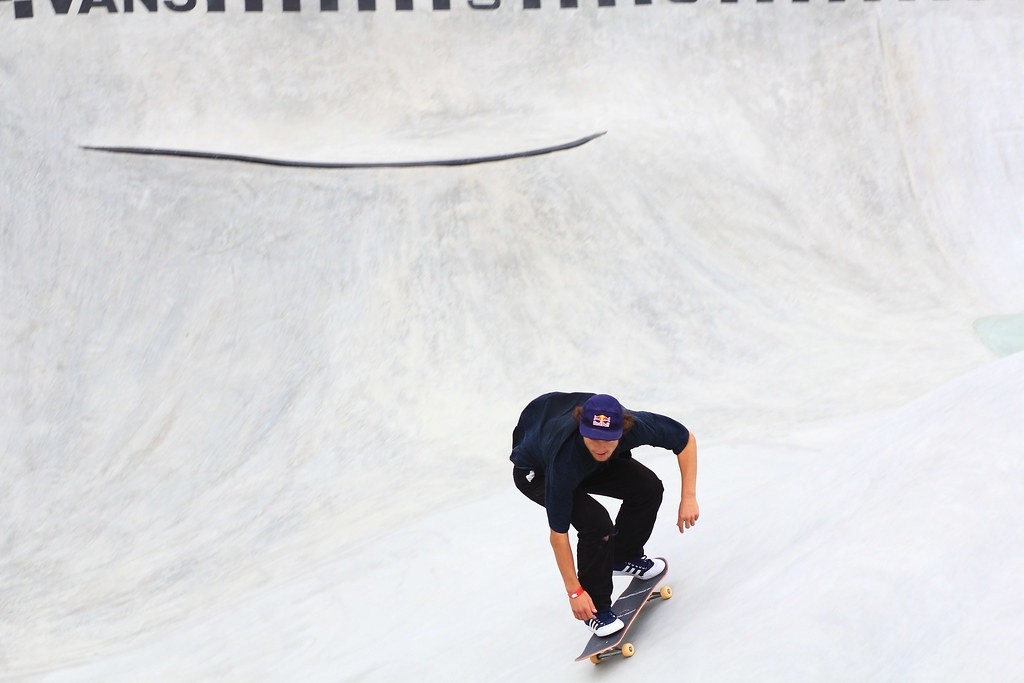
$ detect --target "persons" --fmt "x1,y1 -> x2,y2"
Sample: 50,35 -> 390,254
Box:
509,391 -> 699,636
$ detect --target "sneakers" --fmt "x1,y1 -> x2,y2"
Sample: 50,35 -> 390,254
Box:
584,609 -> 625,637
611,554 -> 666,581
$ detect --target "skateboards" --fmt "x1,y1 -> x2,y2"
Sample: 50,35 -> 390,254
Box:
576,556 -> 671,663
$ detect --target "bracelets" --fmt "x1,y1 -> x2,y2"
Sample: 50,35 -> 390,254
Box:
568,587 -> 584,599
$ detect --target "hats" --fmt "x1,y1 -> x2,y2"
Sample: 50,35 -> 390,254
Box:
578,394 -> 624,441
